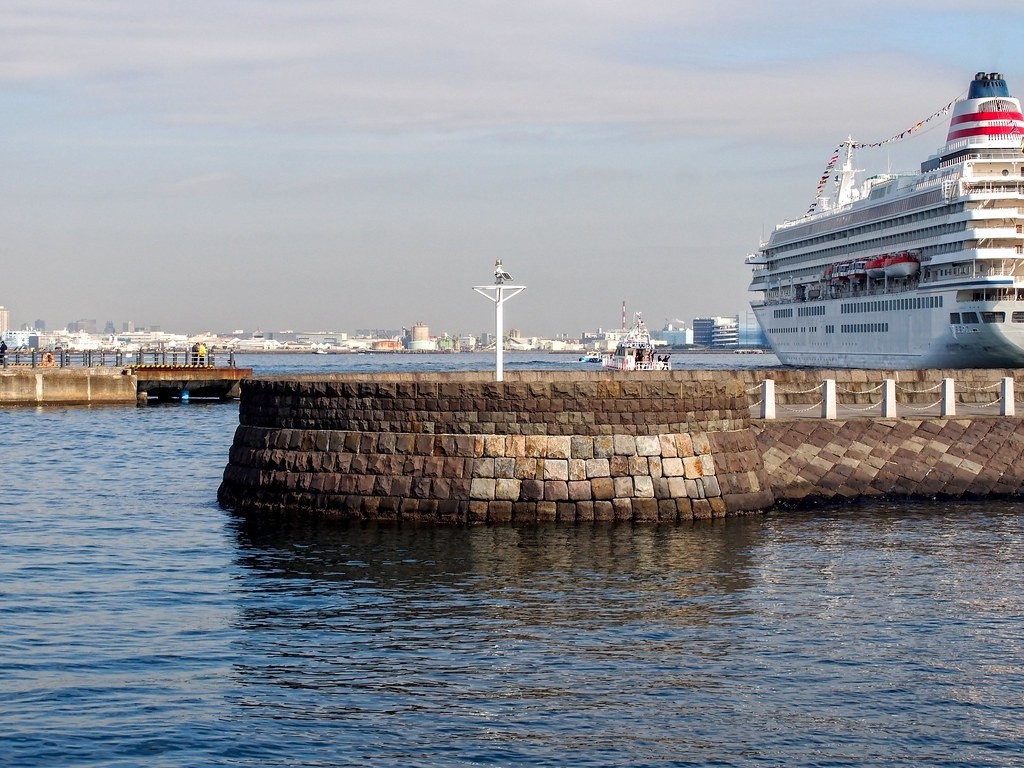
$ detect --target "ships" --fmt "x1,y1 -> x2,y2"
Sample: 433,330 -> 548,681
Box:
746,69 -> 1024,368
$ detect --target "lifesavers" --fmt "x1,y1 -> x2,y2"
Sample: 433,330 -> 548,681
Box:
42,353 -> 54,367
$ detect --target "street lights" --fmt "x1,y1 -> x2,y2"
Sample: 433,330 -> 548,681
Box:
471,256 -> 528,381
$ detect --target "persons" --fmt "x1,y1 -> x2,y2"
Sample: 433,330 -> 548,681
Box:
0,341 -> 7,365
635,350 -> 671,370
192,342 -> 206,365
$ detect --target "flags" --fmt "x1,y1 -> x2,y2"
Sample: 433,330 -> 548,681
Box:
995,96 -> 1024,153
805,144 -> 844,214
852,98 -> 957,149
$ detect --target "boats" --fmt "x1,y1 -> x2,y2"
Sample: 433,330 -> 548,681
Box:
578,351 -> 602,362
884,252 -> 920,279
846,259 -> 867,284
862,255 -> 887,280
832,261 -> 851,284
600,311 -> 673,371
